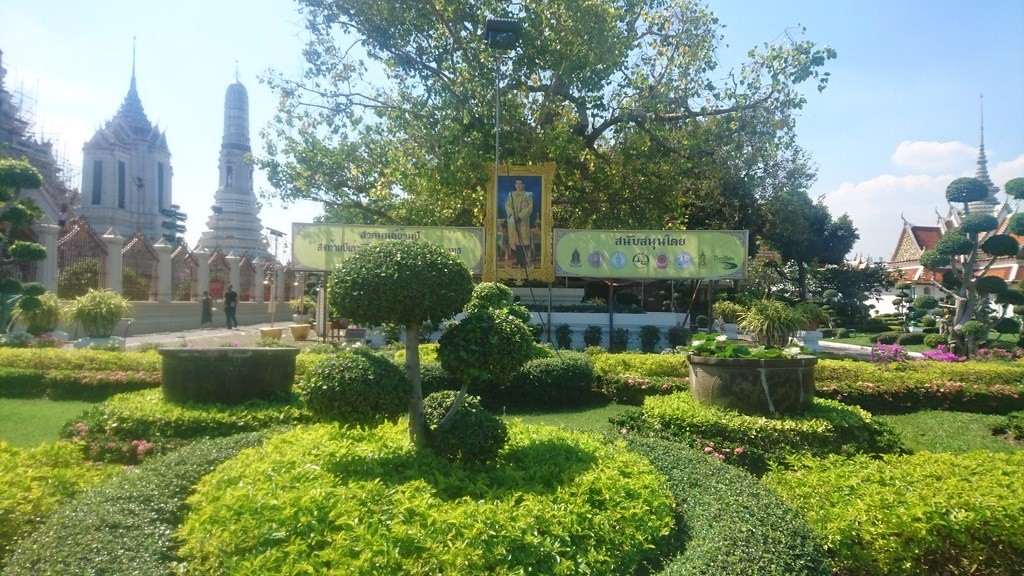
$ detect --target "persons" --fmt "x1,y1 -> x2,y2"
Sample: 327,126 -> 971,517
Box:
200,290 -> 214,330
223,285 -> 238,329
